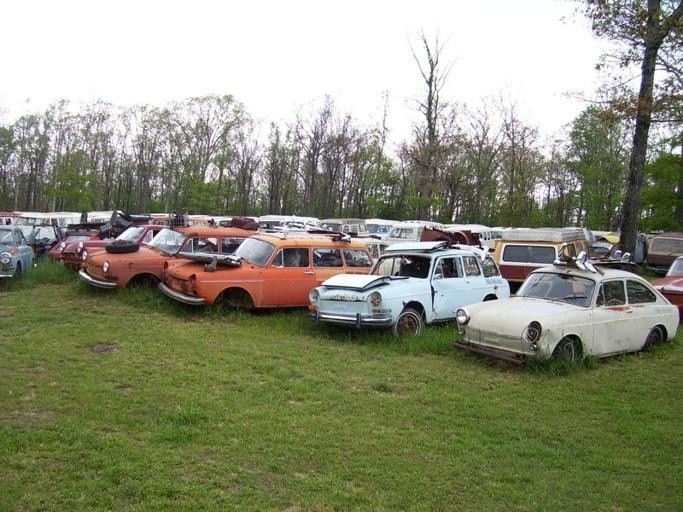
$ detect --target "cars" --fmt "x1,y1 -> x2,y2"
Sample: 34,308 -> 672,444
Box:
157,233 -> 376,316
648,256 -> 683,318
452,263 -> 680,376
308,241 -> 510,339
0,207 -> 683,288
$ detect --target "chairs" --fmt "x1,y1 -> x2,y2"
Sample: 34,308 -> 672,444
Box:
284,249 -> 302,266
550,281 -> 615,307
